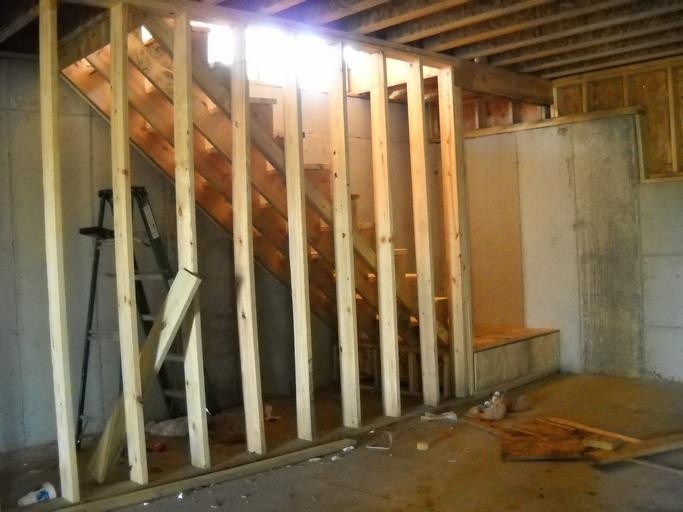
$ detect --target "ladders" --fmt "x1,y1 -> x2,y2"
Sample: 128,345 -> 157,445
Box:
77,186 -> 220,453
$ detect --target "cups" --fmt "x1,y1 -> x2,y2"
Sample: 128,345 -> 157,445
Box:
17,481 -> 56,508
